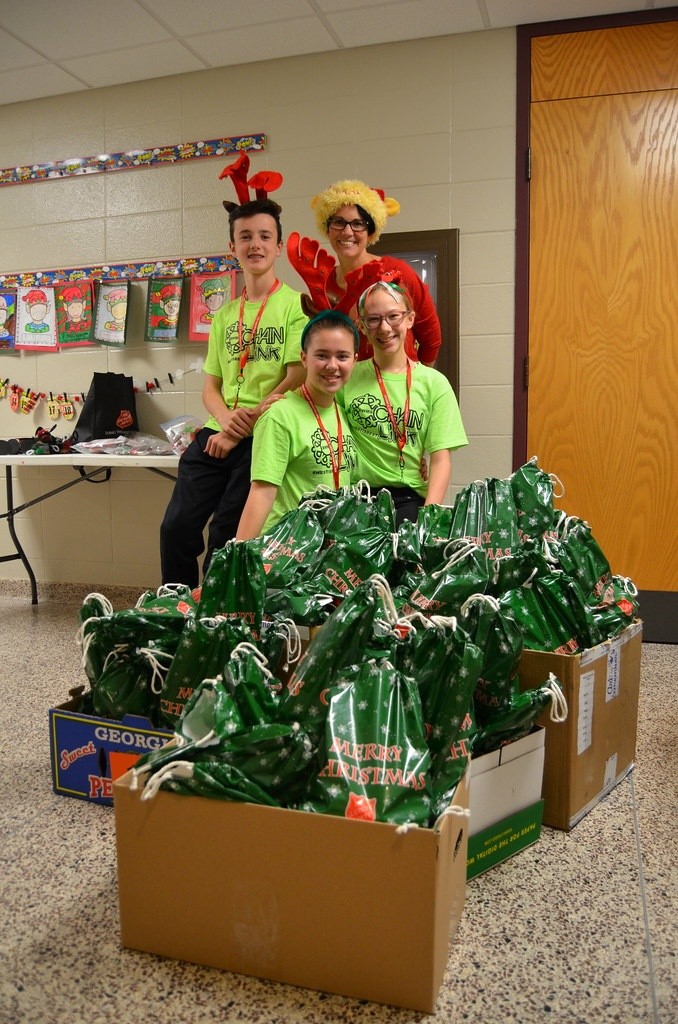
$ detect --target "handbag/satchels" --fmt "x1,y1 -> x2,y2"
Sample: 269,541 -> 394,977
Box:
67,371 -> 139,443
73,456 -> 644,830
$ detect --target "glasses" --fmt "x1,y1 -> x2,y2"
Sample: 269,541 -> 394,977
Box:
326,218 -> 370,231
361,309 -> 411,330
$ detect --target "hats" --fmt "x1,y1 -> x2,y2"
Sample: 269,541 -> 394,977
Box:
311,179 -> 400,248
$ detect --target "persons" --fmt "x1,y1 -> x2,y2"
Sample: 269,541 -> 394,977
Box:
160,198 -> 310,597
236,282 -> 469,587
310,179 -> 442,369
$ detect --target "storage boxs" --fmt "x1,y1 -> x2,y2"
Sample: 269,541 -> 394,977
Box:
515,614 -> 645,834
111,762 -> 472,1016
48,685 -> 177,808
466,723 -> 547,886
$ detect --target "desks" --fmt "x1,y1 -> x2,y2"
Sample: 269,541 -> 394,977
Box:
0,454 -> 182,605
